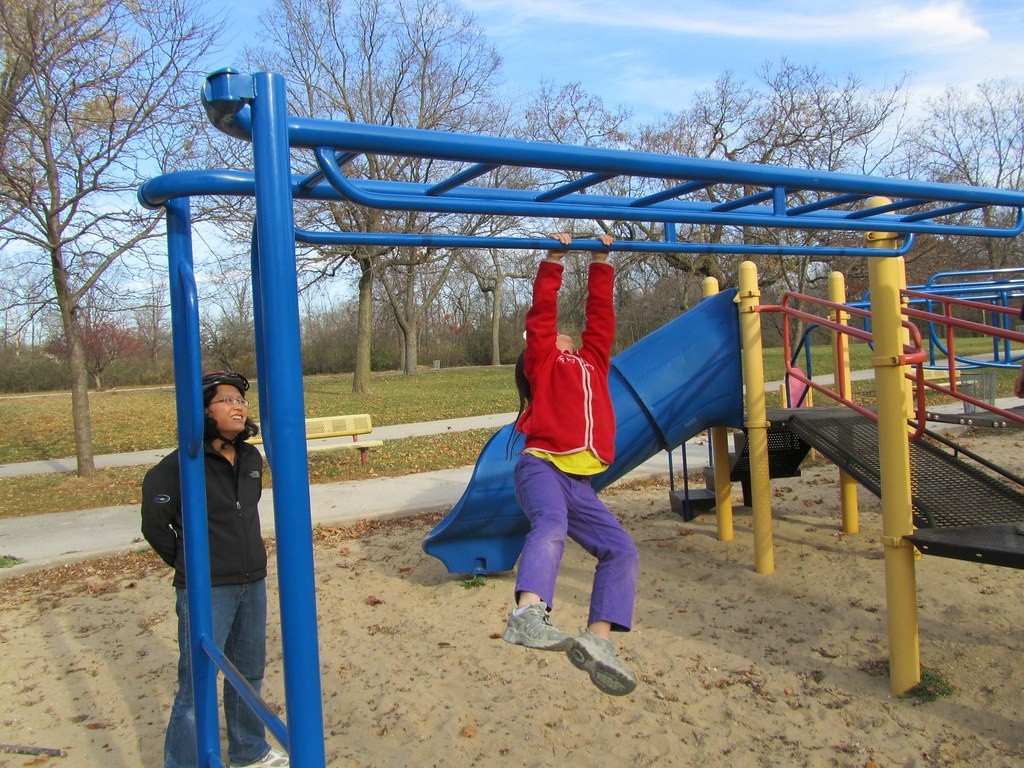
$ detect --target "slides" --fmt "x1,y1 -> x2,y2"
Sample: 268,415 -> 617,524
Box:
422,287 -> 745,576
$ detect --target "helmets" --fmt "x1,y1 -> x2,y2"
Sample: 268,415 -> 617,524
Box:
202,369 -> 250,397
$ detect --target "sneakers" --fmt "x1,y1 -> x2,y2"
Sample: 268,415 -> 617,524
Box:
503,602 -> 575,652
567,626 -> 638,696
230,747 -> 290,768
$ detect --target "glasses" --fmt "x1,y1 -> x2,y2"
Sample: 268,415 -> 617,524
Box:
209,397 -> 249,406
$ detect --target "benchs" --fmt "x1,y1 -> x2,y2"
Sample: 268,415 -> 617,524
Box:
244,413 -> 384,466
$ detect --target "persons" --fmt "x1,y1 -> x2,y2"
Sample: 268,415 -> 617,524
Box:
141,371 -> 289,768
503,233 -> 639,697
